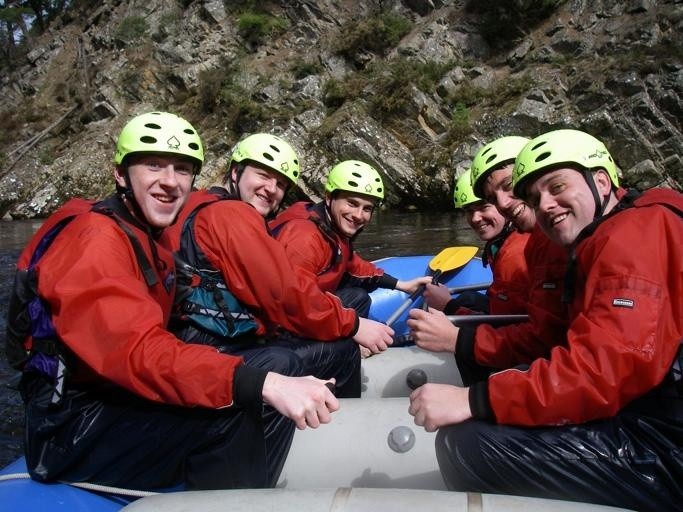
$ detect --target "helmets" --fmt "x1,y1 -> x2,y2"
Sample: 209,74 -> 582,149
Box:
324,159 -> 385,204
453,168 -> 485,210
511,126 -> 621,203
112,111 -> 204,176
469,136 -> 533,200
226,132 -> 300,188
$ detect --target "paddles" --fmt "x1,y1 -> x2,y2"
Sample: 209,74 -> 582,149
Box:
359,246 -> 480,357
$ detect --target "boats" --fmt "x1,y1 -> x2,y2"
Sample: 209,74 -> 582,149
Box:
0,252 -> 653,512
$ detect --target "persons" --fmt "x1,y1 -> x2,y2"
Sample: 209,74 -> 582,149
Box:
20,110 -> 339,491
425,170 -> 532,322
406,136 -> 574,367
166,134 -> 395,398
409,127 -> 682,512
269,161 -> 432,349
